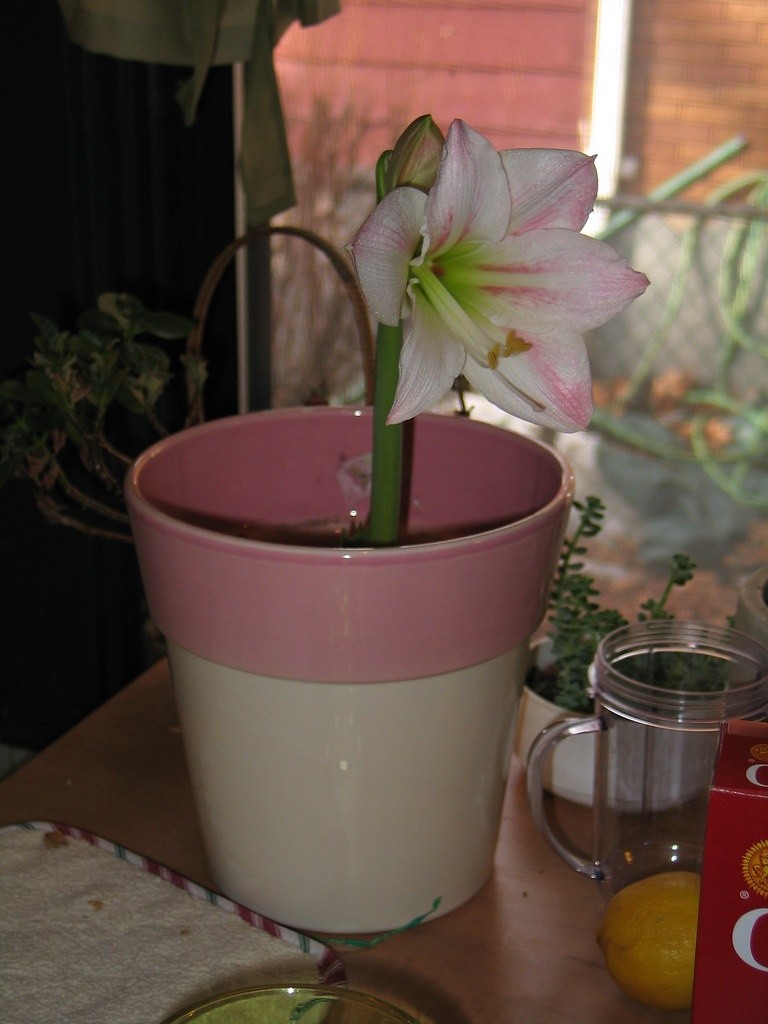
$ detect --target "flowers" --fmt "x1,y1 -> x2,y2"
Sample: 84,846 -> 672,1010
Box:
6,99 -> 652,581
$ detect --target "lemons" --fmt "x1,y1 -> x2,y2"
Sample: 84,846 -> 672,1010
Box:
593,869 -> 703,1010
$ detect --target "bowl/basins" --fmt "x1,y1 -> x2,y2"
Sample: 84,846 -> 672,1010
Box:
158,985 -> 414,1024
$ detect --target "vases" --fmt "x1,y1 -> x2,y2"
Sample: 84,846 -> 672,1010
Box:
138,405 -> 573,934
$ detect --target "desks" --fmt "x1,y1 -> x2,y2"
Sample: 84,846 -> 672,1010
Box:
0,653 -> 704,1024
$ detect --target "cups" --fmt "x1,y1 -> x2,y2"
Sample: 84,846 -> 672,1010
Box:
524,620 -> 768,899
